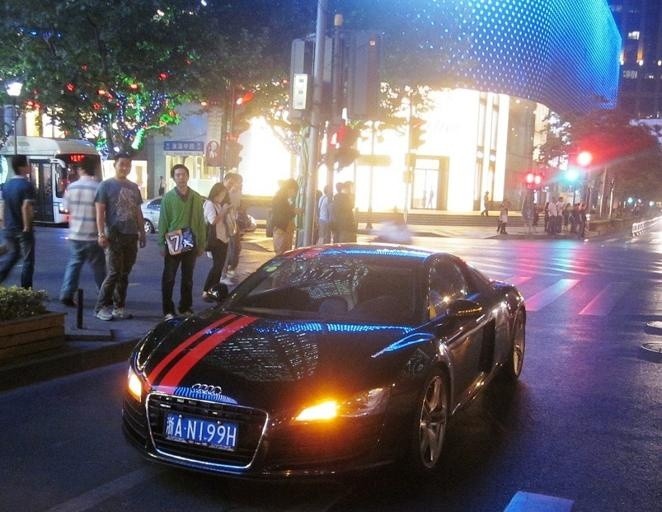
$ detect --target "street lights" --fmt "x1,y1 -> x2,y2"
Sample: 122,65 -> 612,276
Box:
571,150 -> 591,205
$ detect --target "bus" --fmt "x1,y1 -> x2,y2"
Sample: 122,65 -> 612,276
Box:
2,135 -> 103,227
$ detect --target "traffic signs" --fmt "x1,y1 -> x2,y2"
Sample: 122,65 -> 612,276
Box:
162,140 -> 204,154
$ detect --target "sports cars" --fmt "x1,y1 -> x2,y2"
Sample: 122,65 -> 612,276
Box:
122,242 -> 528,476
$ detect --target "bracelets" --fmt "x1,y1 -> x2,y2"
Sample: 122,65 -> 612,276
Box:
97,231 -> 105,238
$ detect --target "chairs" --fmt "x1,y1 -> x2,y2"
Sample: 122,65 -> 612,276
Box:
318,295 -> 347,316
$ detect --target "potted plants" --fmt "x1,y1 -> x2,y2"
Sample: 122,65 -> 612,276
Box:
0,284 -> 70,362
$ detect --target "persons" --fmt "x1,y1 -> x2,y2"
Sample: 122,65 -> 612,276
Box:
95,153 -> 147,320
159,165 -> 207,321
369,212 -> 415,245
202,172 -> 357,303
58,155 -> 113,308
427,187 -> 434,209
481,190 -> 587,238
0,153 -> 37,298
157,175 -> 167,197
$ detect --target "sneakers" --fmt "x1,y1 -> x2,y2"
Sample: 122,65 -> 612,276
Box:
164,314 -> 174,322
112,306 -> 132,319
202,270 -> 238,301
93,307 -> 112,321
62,297 -> 77,307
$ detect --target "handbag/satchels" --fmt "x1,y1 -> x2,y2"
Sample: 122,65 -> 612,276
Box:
165,227 -> 195,255
265,209 -> 273,237
204,222 -> 216,251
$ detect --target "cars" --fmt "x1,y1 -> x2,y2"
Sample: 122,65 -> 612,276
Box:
138,195 -> 256,234
